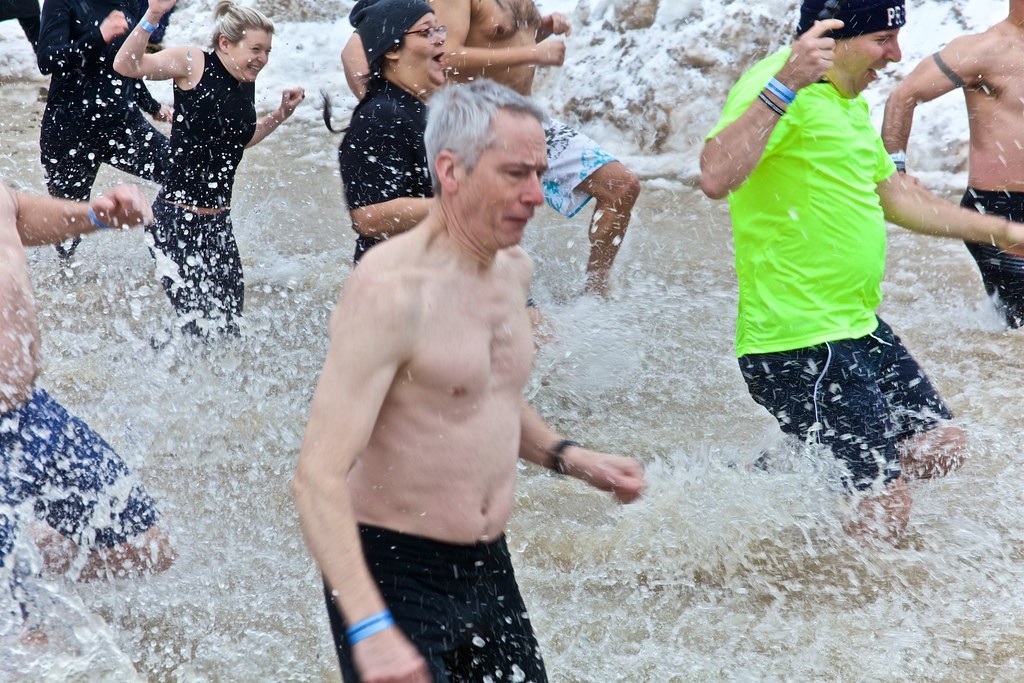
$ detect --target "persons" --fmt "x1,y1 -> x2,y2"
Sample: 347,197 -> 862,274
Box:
699,0 -> 966,547
427,0 -> 644,296
321,1 -> 554,355
37,2 -> 180,269
0,1 -> 44,54
880,1 -> 1024,334
293,79 -> 650,681
0,171 -> 180,642
110,0 -> 307,354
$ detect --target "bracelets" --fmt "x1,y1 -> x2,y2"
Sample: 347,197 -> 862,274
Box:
890,153 -> 908,174
548,440 -> 581,479
760,76 -> 799,119
88,208 -> 115,234
345,609 -> 397,649
139,19 -> 160,35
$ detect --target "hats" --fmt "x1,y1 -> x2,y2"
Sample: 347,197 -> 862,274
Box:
796,0 -> 907,40
348,0 -> 435,68
